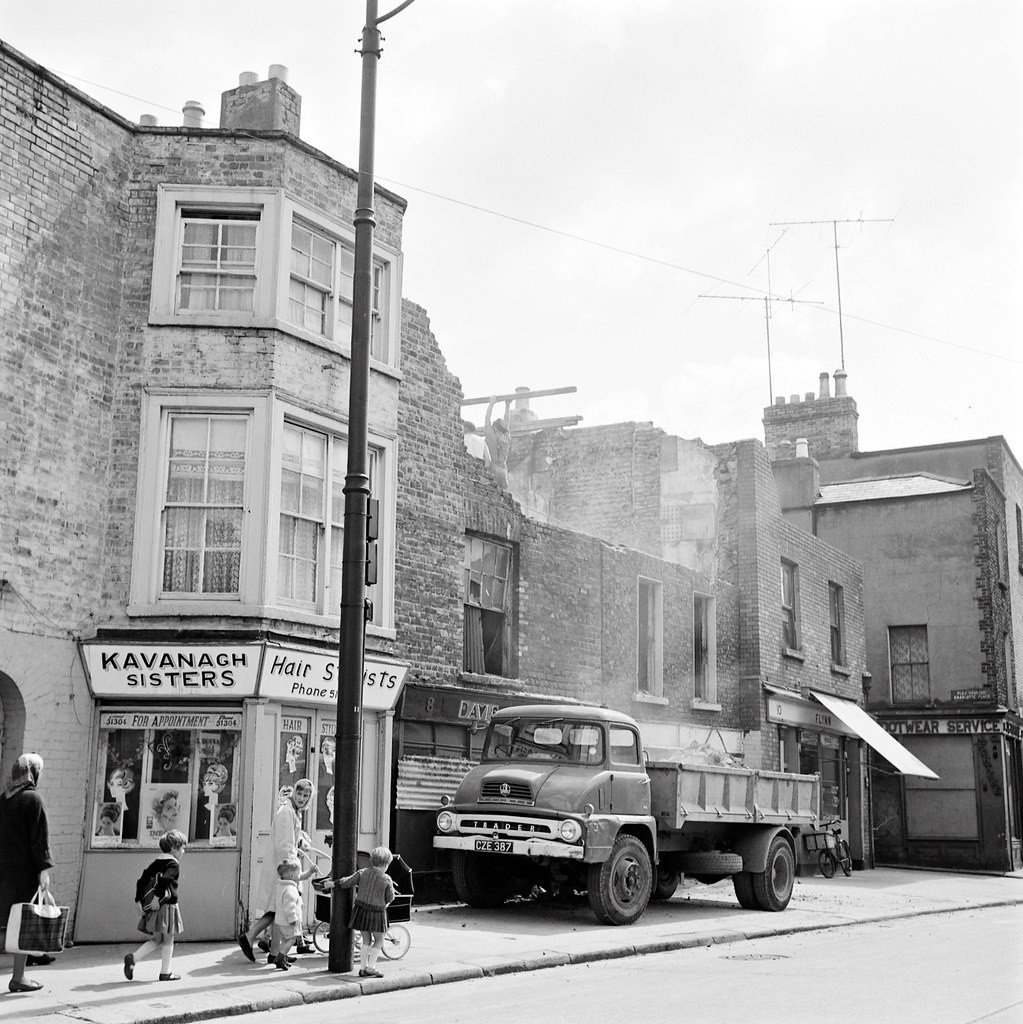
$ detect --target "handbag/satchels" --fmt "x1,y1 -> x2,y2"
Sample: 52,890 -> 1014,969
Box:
4,885 -> 70,957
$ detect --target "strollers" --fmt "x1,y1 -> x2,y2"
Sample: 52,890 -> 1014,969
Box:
298,836 -> 416,961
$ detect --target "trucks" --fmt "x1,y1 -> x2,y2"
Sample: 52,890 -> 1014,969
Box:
431,706 -> 826,928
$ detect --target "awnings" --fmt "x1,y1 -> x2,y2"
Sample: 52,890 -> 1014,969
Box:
801,688 -> 940,780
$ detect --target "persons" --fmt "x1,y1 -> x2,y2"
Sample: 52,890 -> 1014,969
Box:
214,805 -> 236,836
124,830 -> 186,981
324,847 -> 394,978
273,859 -> 304,971
237,779 -> 314,963
0,753 -> 55,992
153,790 -> 181,829
258,785 -> 313,951
95,804 -> 120,837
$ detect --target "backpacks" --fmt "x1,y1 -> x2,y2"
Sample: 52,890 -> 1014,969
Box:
139,871 -> 172,911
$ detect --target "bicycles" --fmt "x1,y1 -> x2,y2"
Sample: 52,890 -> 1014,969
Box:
818,819 -> 853,879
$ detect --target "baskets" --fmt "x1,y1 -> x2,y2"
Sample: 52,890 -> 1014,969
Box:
804,832 -> 837,852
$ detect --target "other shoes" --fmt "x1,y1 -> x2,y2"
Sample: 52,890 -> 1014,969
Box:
268,954 -> 297,964
9,980 -> 44,991
159,972 -> 181,980
366,969 -> 384,978
25,954 -> 57,966
237,933 -> 256,963
297,945 -> 315,953
276,961 -> 292,968
124,953 -> 135,982
275,953 -> 288,971
359,969 -> 367,978
257,940 -> 271,953
292,938 -> 313,945
